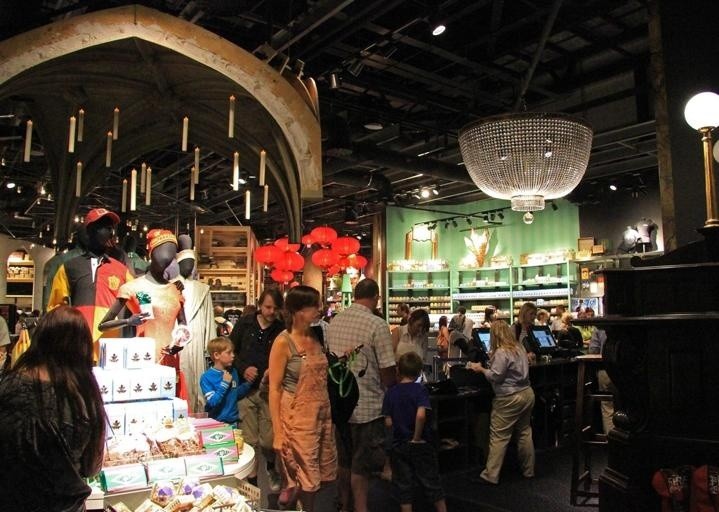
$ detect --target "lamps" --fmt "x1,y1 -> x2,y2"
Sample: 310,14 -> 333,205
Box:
363,111 -> 387,134
458,111 -> 595,224
463,227 -> 491,267
433,186 -> 441,196
682,91 -> 719,231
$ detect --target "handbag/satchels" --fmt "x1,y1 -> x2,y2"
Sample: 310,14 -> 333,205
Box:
325,347 -> 359,428
0,369 -> 91,512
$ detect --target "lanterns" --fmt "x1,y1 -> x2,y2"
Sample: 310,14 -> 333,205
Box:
254,227 -> 367,285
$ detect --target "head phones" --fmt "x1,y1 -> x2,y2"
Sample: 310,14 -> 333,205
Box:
357,352 -> 370,381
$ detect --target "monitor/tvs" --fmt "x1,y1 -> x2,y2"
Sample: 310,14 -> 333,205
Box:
473,328 -> 497,355
528,325 -> 556,351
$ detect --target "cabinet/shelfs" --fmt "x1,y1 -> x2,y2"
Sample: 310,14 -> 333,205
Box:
323,268 -> 362,317
513,252 -> 576,330
193,224 -> 266,314
386,260 -> 451,328
5,259 -> 36,312
455,263 -> 512,333
579,247 -> 667,299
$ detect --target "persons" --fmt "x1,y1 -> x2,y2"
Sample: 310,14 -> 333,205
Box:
0,305 -> 114,512
200,281 -> 626,512
46,209 -> 216,414
617,225 -> 641,254
634,217 -> 653,243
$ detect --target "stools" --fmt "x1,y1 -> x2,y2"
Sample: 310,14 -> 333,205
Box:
570,353 -> 615,508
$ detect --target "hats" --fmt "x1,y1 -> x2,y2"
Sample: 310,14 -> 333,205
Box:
145,229 -> 178,258
84,208 -> 121,226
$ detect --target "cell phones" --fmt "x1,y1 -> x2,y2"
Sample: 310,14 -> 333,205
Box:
336,342 -> 364,359
